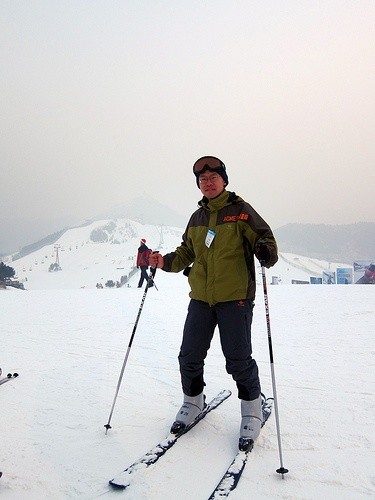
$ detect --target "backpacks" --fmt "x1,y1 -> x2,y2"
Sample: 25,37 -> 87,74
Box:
144,249 -> 152,263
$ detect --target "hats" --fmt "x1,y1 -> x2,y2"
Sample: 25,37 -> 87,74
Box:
193,156 -> 229,189
140,239 -> 146,244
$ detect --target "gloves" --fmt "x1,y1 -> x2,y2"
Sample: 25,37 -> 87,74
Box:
257,243 -> 270,263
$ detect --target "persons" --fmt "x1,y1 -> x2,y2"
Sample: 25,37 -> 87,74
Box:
150,156 -> 278,442
137,239 -> 149,288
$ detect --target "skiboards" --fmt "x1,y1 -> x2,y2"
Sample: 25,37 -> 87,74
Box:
109,388 -> 273,500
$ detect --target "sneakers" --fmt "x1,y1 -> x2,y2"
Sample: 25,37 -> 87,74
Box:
176,393 -> 206,425
239,394 -> 263,439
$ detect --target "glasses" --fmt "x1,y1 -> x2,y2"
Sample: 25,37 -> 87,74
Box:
197,173 -> 221,185
193,156 -> 226,175
